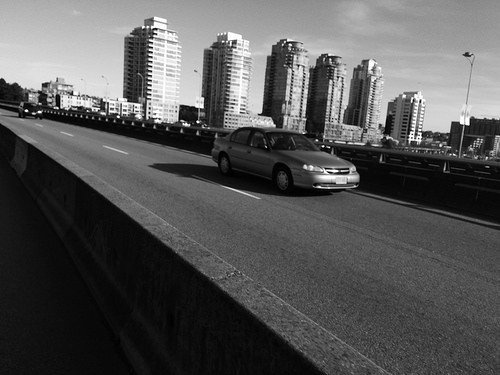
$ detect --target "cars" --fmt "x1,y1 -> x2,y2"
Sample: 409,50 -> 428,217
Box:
18,101 -> 43,120
210,126 -> 361,195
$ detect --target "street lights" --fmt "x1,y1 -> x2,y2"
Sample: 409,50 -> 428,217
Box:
194,69 -> 202,125
457,52 -> 476,159
137,72 -> 144,120
102,75 -> 108,116
